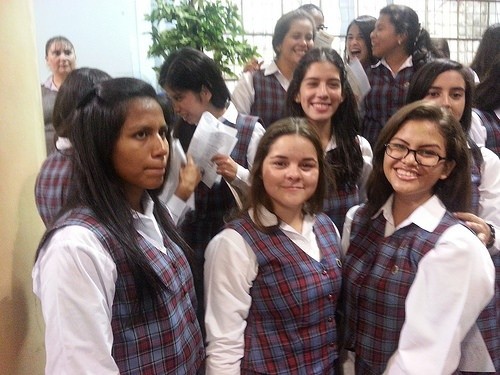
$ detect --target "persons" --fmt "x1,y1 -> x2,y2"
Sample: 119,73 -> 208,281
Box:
30,2 -> 500,375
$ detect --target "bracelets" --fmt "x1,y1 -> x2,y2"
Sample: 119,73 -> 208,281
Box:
485,223 -> 495,248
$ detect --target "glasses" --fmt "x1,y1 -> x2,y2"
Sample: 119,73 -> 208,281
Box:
384,143 -> 446,167
316,25 -> 327,32
